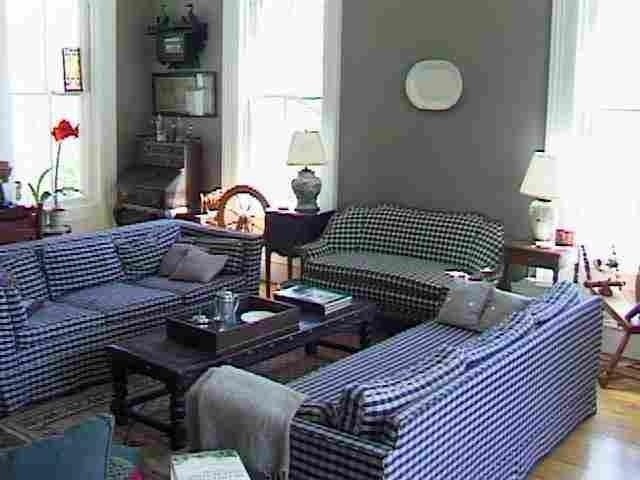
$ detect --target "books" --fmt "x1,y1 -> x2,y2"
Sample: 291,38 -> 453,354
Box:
272,279 -> 353,315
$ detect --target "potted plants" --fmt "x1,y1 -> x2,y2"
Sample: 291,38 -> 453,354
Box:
26,166 -> 52,229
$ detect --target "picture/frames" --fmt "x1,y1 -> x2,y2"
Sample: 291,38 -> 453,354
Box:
63,47 -> 83,92
150,69 -> 218,118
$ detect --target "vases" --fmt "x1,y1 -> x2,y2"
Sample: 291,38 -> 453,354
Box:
48,208 -> 66,229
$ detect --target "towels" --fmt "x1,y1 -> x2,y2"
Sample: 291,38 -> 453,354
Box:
184,364 -> 309,480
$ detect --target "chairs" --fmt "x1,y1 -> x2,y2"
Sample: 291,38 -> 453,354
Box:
587,278 -> 640,374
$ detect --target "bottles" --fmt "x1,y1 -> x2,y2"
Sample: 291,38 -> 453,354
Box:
151,111 -> 195,143
554,228 -> 576,247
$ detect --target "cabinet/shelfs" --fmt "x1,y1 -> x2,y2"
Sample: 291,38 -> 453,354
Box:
113,138 -> 203,226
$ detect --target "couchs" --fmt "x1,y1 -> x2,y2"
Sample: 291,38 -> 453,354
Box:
292,203 -> 506,318
183,280 -> 605,480
0,218 -> 266,422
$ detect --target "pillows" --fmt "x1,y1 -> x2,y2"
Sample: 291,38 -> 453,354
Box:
434,278 -> 497,332
0,225 -> 243,331
339,279 -> 582,437
479,286 -> 536,332
297,395 -> 339,427
0,412 -> 114,480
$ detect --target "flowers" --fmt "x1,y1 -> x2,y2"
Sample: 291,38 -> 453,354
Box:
50,120 -> 79,211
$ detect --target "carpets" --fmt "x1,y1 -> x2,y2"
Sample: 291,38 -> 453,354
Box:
599,353 -> 640,389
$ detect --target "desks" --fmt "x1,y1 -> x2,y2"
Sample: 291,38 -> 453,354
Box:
264,208 -> 335,297
0,206 -> 41,245
507,239 -> 580,290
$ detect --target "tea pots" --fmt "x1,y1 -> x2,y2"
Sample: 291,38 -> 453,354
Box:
209,286 -> 240,329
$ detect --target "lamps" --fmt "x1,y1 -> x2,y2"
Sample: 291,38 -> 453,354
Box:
284,132 -> 328,212
520,152 -> 569,248
